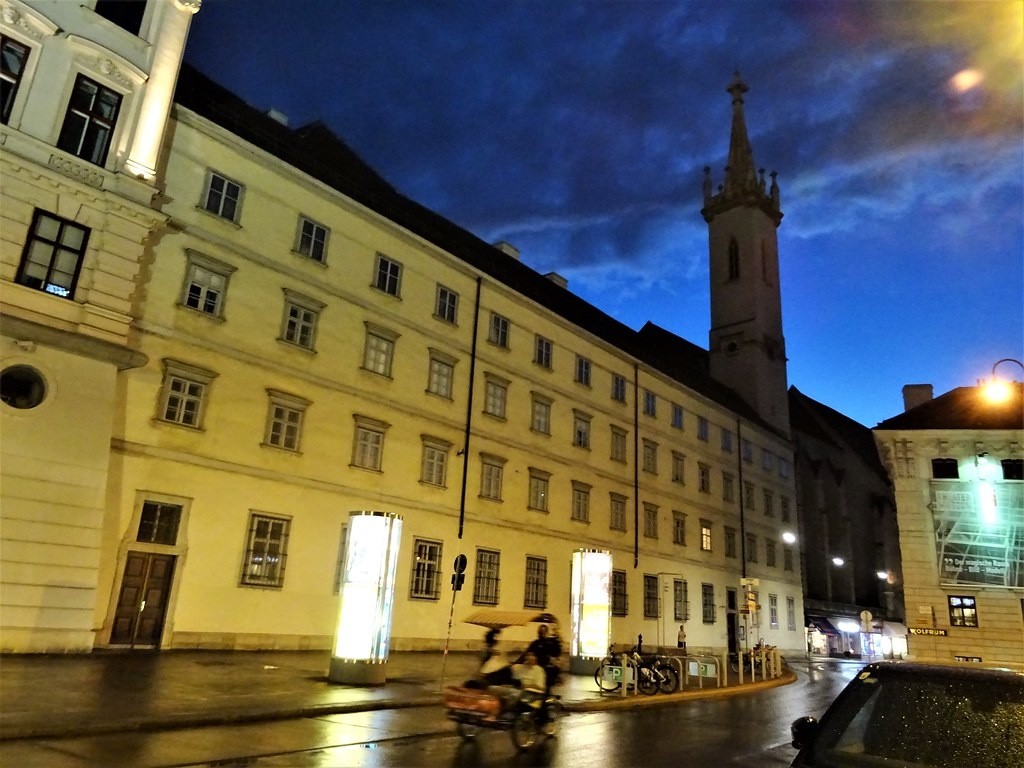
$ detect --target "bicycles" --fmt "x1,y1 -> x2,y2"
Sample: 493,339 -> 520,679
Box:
731,643 -> 787,677
594,643 -> 679,696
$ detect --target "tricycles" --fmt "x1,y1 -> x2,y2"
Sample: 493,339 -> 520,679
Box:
446,653 -> 562,752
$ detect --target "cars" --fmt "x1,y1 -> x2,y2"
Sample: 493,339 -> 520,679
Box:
788,658 -> 1024,768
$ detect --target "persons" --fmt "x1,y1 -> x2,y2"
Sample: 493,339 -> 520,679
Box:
678,625 -> 686,649
510,624 -> 562,716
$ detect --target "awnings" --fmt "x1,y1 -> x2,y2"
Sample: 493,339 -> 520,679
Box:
806,616 -> 908,639
